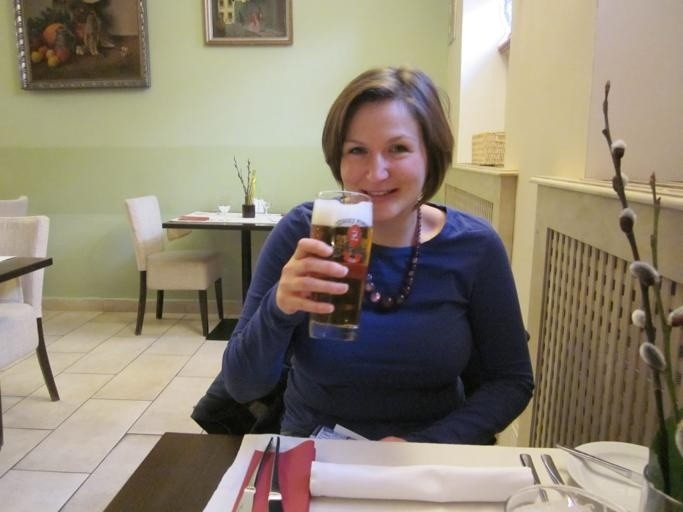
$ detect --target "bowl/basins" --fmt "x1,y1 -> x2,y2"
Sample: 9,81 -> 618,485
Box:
505,484 -> 627,512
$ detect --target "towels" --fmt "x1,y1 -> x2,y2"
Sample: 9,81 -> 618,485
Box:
178,215 -> 209,222
309,460 -> 532,503
233,440 -> 315,512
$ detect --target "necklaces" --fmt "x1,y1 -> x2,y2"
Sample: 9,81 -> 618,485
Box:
361,206 -> 421,308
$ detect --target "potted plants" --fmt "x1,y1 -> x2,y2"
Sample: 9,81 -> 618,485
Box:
234,154 -> 258,217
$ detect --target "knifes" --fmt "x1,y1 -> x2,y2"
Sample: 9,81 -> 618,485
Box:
235,436 -> 273,512
267,436 -> 285,512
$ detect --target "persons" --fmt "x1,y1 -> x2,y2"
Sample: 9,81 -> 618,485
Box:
190,66 -> 535,443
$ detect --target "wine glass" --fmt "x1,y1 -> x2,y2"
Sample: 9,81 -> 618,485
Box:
261,199 -> 272,217
216,201 -> 233,220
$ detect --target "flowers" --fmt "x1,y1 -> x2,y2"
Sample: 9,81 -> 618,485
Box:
599,81 -> 683,512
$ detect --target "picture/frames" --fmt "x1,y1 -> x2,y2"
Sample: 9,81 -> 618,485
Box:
204,0 -> 294,46
14,0 -> 151,91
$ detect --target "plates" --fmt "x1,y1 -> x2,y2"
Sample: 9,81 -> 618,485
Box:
567,441 -> 650,489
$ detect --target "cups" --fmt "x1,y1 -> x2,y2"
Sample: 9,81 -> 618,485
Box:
309,192 -> 372,342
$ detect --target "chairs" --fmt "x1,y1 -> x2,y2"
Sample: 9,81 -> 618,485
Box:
124,195 -> 225,336
1,195 -> 27,217
0,216 -> 60,447
